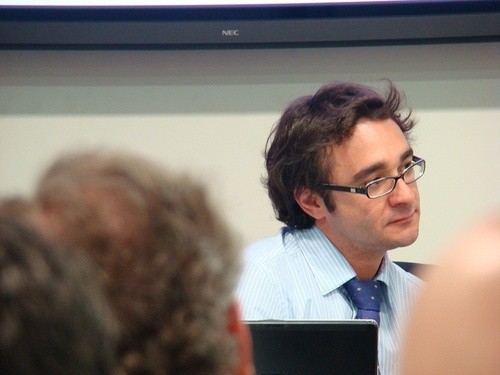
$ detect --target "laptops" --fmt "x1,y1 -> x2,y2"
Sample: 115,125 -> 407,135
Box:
242,318 -> 378,375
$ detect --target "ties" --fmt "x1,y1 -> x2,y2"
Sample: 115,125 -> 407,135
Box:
344,278 -> 385,327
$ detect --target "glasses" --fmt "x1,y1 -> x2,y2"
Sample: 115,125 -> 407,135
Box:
319,155 -> 426,200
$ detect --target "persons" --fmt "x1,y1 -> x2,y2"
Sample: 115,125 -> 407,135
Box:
232,78 -> 431,375
1,151 -> 259,375
